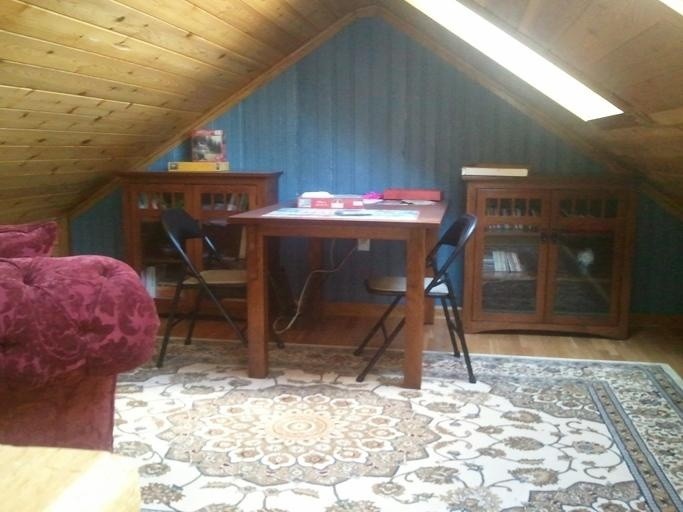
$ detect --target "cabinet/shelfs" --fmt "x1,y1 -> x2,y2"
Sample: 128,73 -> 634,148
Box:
119,171 -> 286,322
461,172 -> 637,340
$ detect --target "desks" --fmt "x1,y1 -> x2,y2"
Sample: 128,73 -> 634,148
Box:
228,196 -> 452,389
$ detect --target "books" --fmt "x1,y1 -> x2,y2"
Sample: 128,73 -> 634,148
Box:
480,206 -> 569,277
137,190 -> 248,298
460,161 -> 530,177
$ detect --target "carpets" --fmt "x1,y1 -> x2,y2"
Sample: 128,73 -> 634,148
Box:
0,335 -> 683,512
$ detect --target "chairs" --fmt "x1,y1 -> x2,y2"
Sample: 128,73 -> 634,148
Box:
0,218 -> 160,453
352,213 -> 479,385
155,212 -> 284,369
